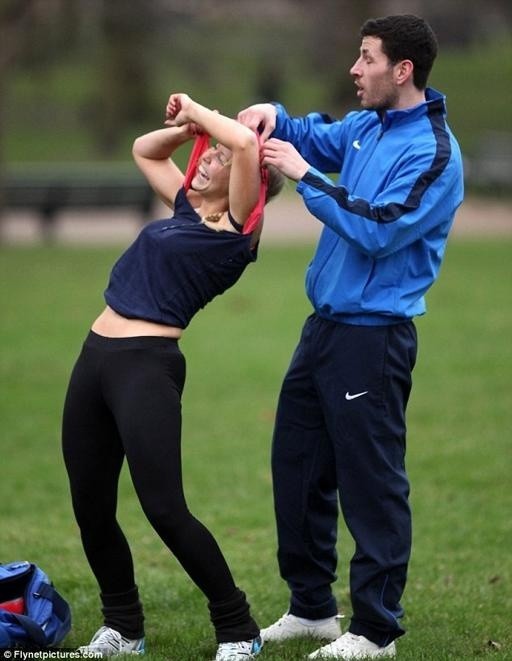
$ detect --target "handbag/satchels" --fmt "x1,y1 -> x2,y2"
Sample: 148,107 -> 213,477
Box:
0,561 -> 71,649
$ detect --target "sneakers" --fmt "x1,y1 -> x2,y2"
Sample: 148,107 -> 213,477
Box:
76,627 -> 146,658
260,612 -> 346,641
306,631 -> 397,660
215,634 -> 263,661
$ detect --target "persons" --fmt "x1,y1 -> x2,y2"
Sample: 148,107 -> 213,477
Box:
236,13 -> 464,661
59,92 -> 286,661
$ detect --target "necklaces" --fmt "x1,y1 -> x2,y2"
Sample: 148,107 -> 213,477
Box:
199,210 -> 226,224
